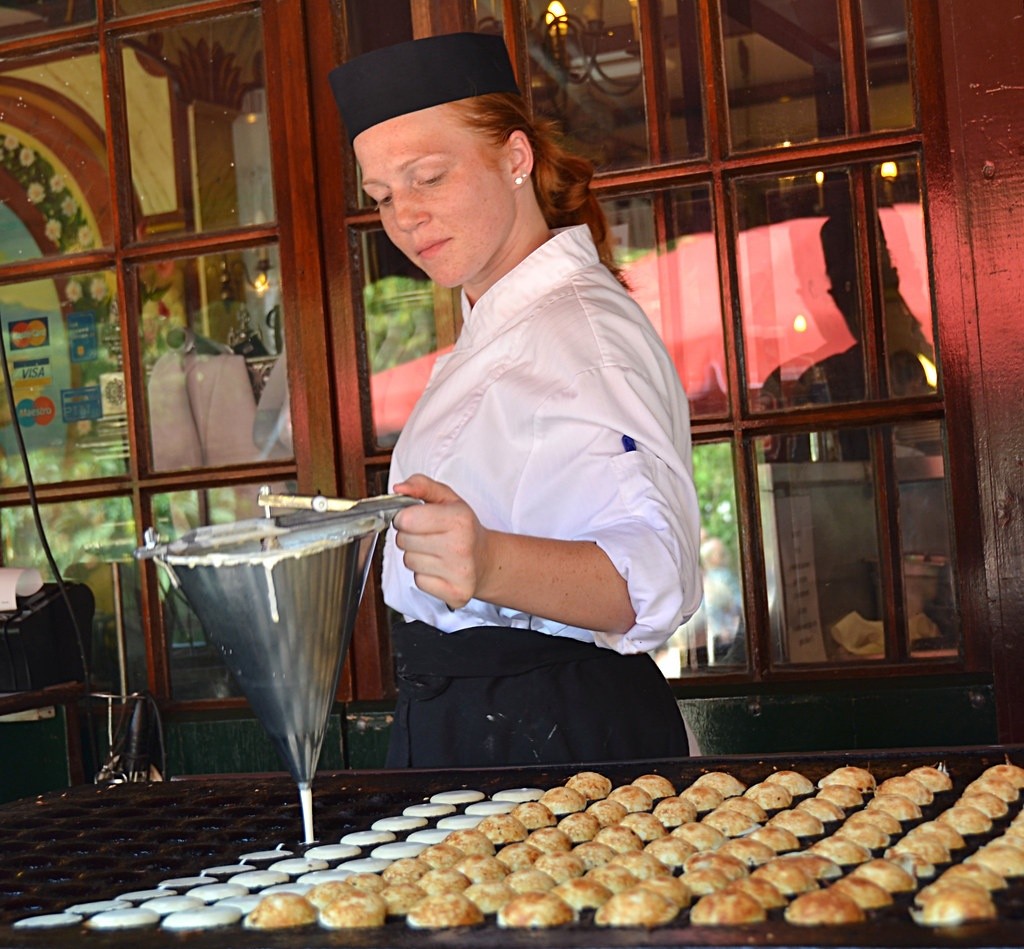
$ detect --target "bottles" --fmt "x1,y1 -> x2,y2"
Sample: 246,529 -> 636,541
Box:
808,365 -> 843,462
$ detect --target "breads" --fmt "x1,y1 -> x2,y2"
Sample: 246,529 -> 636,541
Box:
241,764 -> 1024,928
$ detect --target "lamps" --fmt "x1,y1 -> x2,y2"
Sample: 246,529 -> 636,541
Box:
527,0 -> 643,118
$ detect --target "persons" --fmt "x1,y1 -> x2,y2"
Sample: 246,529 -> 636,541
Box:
326,34 -> 704,768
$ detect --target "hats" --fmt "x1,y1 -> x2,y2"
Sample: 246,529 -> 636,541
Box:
329,32 -> 523,145
